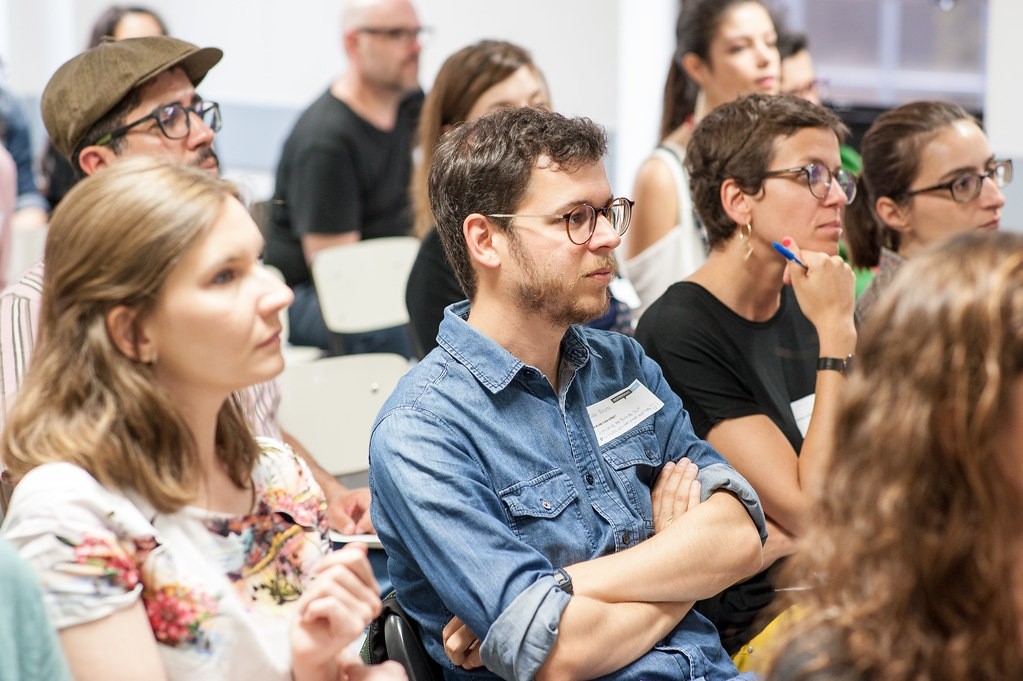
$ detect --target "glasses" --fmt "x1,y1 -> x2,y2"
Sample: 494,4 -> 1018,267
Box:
908,159 -> 1014,204
96,101 -> 223,144
762,160 -> 859,206
486,197 -> 635,245
356,25 -> 423,43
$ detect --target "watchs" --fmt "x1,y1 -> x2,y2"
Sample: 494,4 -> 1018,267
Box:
816,354 -> 854,379
554,566 -> 574,596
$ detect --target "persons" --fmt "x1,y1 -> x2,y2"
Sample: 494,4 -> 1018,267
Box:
2,1 -> 1023,681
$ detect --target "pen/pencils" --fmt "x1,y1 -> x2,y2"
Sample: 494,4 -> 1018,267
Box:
772,241 -> 809,271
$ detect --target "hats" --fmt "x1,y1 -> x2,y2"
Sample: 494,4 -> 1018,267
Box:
41,34 -> 224,162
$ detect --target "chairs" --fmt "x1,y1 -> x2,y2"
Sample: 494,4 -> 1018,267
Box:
380,603 -> 442,681
312,235 -> 425,363
277,352 -> 412,480
